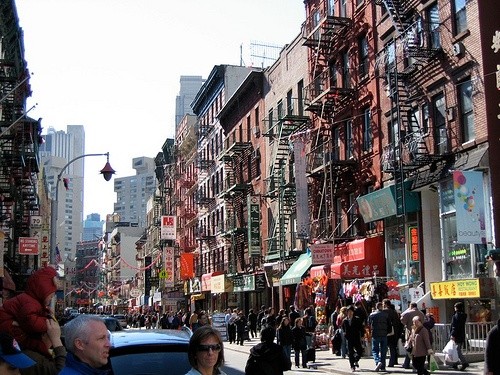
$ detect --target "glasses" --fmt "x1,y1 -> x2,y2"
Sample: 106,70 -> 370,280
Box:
198,344 -> 221,352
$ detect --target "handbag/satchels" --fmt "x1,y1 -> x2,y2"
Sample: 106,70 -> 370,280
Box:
404,338 -> 413,353
429,353 -> 439,372
442,339 -> 462,367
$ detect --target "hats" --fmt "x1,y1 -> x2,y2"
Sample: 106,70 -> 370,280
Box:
0,330 -> 38,370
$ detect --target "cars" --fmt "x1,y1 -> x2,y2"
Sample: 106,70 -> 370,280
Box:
104,326 -> 198,375
112,314 -> 127,328
100,317 -> 122,333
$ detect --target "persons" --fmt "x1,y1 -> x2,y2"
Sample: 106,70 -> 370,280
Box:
449,301 -> 469,371
387,303 -> 400,367
342,308 -> 364,371
245,325 -> 292,375
419,309 -> 435,370
292,318 -> 308,369
368,301 -> 392,372
57,313 -> 111,375
407,315 -> 434,375
382,299 -> 400,365
0,265 -> 68,375
400,301 -> 425,369
123,300 -> 367,362
184,326 -> 228,374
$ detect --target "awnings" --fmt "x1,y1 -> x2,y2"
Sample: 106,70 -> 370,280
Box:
280,252 -> 312,286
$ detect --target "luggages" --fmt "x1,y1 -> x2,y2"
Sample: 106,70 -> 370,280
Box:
306,335 -> 315,363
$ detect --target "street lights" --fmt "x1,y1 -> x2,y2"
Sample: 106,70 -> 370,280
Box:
48,152 -> 116,315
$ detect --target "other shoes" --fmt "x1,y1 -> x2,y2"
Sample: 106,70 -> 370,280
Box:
388,359 -> 398,367
229,334 -> 258,346
402,363 -> 414,369
351,362 -> 360,372
460,362 -> 469,371
450,363 -> 458,369
374,362 -> 387,372
